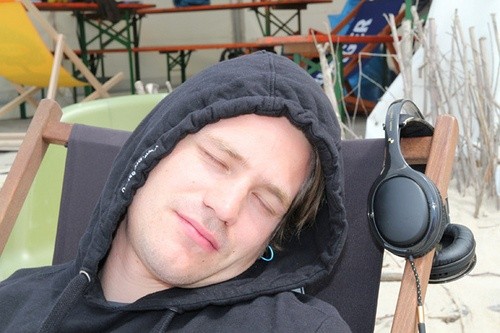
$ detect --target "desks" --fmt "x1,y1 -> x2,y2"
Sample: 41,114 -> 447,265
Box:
136,0 -> 333,93
31,4 -> 155,97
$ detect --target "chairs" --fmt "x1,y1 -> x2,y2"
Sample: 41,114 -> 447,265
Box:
0,98 -> 459,333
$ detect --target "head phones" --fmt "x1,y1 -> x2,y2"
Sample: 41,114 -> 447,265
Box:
366,98 -> 477,284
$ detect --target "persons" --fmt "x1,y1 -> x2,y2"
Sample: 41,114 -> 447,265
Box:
0,50 -> 353,333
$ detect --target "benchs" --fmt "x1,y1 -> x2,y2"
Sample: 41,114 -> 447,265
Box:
38,42 -> 322,105
